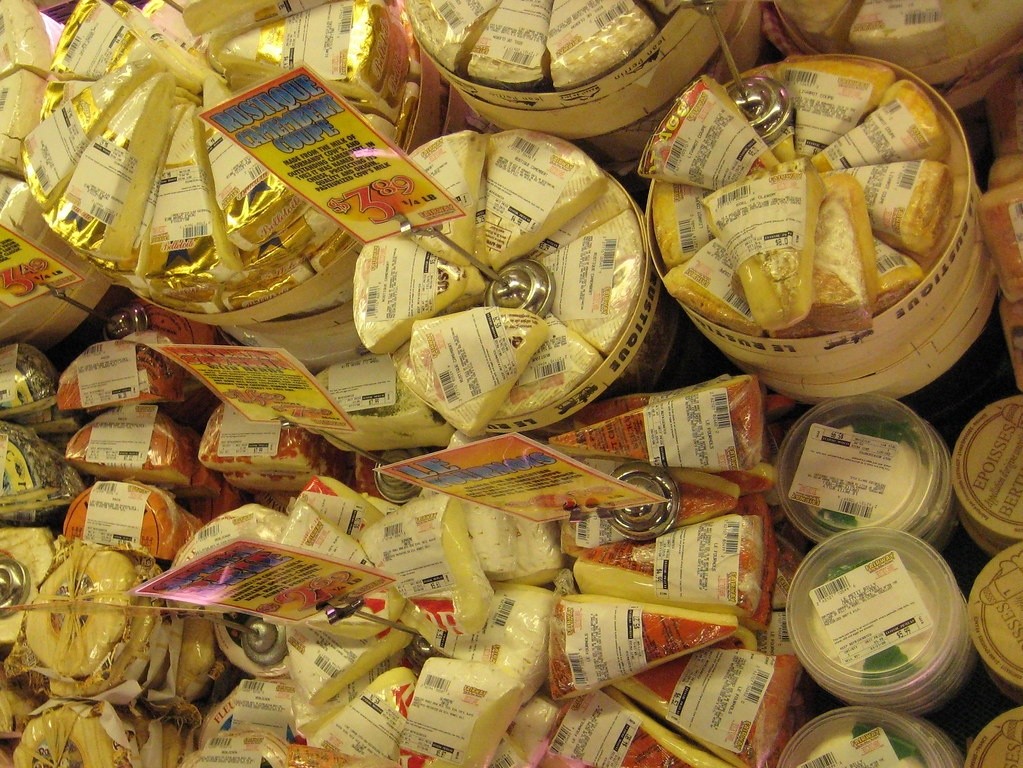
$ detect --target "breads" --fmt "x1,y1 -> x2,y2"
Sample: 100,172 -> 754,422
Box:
0,0 -> 1023,768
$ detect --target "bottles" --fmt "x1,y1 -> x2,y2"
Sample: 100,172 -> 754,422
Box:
779,384 -> 1022,767
